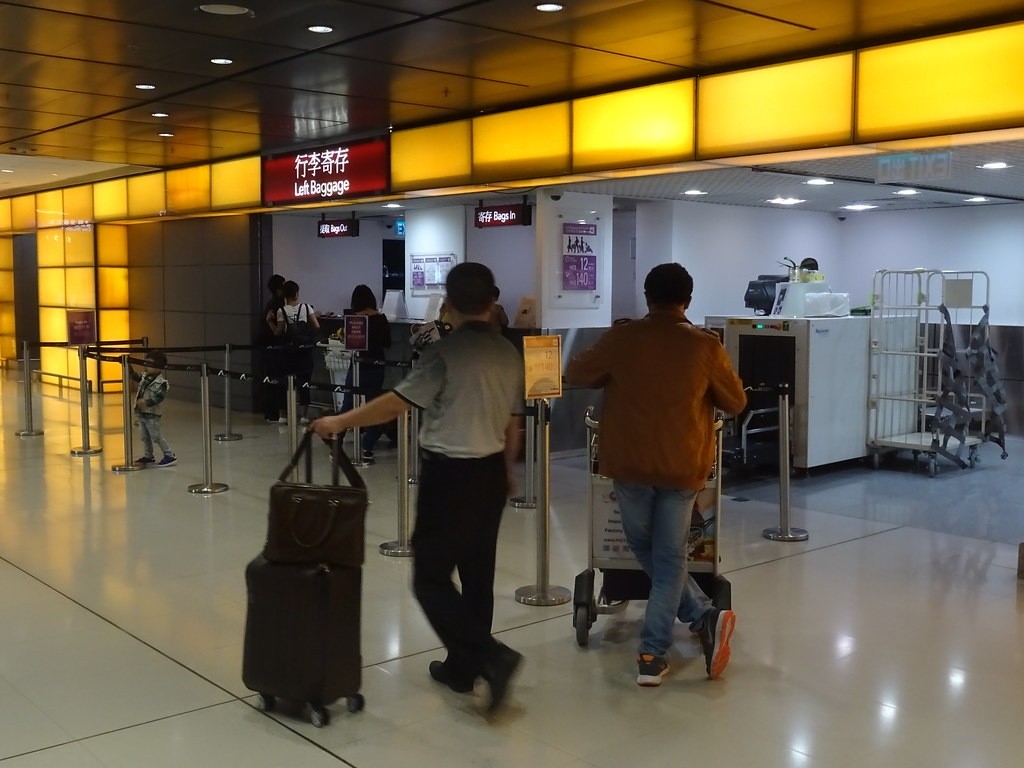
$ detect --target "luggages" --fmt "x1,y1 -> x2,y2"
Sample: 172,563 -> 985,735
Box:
241,426 -> 366,728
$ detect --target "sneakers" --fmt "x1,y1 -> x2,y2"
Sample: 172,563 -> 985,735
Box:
697,609 -> 735,680
157,454 -> 177,467
637,654 -> 671,686
133,456 -> 156,465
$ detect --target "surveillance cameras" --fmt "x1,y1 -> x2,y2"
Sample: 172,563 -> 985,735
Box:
385,224 -> 393,229
838,216 -> 847,221
548,190 -> 565,201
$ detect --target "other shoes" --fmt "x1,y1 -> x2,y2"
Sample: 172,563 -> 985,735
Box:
300,417 -> 310,423
429,660 -> 473,693
264,417 -> 287,423
479,644 -> 521,713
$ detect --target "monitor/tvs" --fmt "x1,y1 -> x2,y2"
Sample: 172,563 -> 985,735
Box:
744,275 -> 791,316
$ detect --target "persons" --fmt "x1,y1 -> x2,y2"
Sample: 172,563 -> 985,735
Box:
309,262 -> 525,722
800,258 -> 818,270
263,274 -> 286,424
128,349 -> 178,467
565,263 -> 747,686
440,297 -> 460,332
266,280 -> 320,425
492,285 -> 509,331
329,284 -> 391,466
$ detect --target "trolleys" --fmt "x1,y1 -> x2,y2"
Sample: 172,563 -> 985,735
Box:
867,267 -> 991,479
570,405 -> 733,646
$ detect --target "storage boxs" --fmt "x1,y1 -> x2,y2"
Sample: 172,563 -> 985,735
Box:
803,293 -> 852,317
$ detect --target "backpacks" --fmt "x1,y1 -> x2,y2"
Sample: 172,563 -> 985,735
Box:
282,303 -> 313,351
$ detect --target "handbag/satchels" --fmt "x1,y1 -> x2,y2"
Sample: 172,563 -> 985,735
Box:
408,319 -> 447,355
262,429 -> 372,569
255,307 -> 284,345
